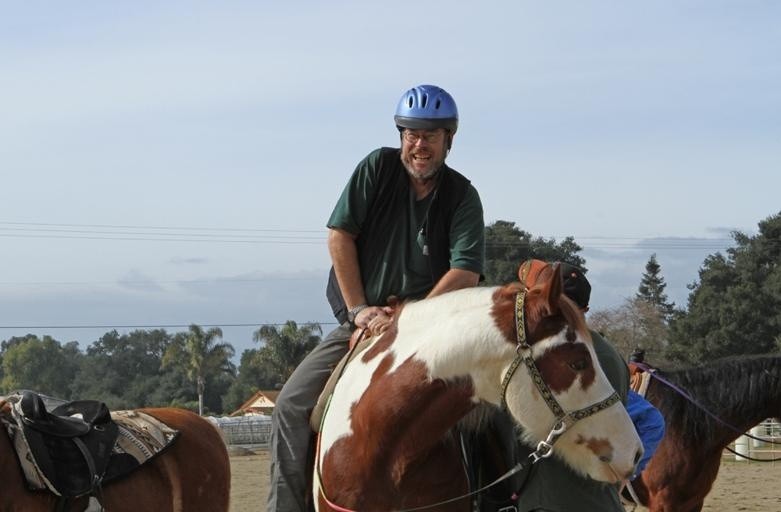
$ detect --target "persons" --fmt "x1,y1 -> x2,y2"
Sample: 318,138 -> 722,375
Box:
494,259 -> 665,512
267,85 -> 519,512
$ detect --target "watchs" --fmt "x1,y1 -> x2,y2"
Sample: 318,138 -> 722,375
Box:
347,303 -> 369,323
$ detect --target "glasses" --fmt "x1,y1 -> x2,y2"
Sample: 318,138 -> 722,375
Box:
400,131 -> 447,144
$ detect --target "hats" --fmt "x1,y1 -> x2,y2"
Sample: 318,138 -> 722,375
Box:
518,258 -> 591,312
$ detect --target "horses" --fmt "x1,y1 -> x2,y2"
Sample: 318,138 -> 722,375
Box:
615,352 -> 781,512
308,262 -> 646,512
0,405 -> 231,512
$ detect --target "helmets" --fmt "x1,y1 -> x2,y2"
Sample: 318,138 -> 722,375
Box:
394,84 -> 459,132
